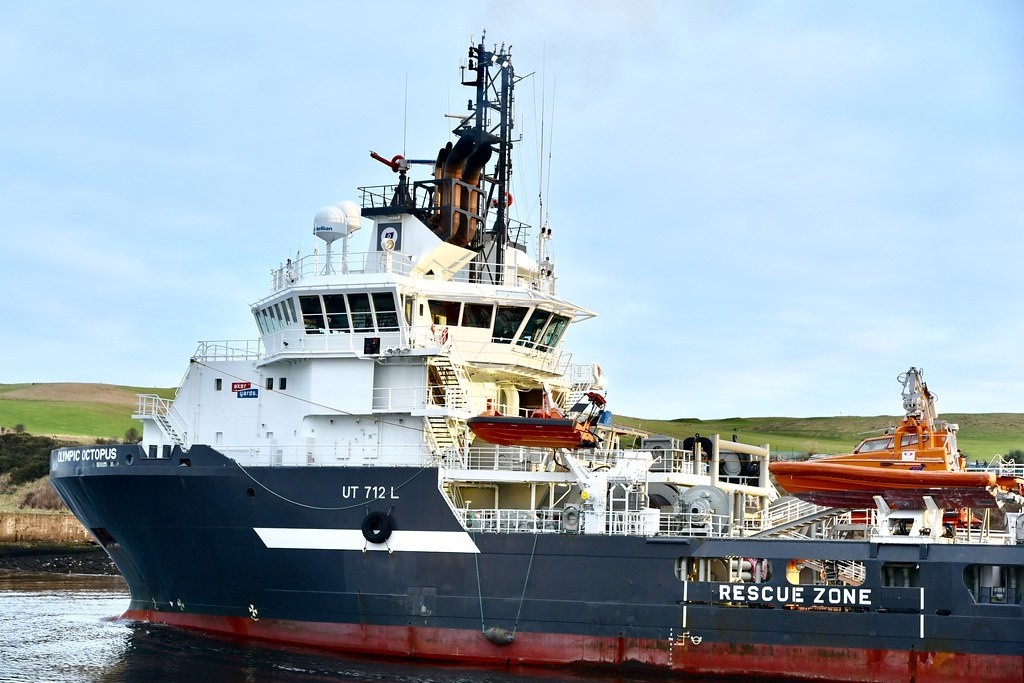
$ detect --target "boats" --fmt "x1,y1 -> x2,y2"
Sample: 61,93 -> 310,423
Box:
465,408 -> 601,450
768,421 -> 1024,529
44,23 -> 1024,683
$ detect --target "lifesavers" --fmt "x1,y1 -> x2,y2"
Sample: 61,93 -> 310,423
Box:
362,512 -> 392,543
